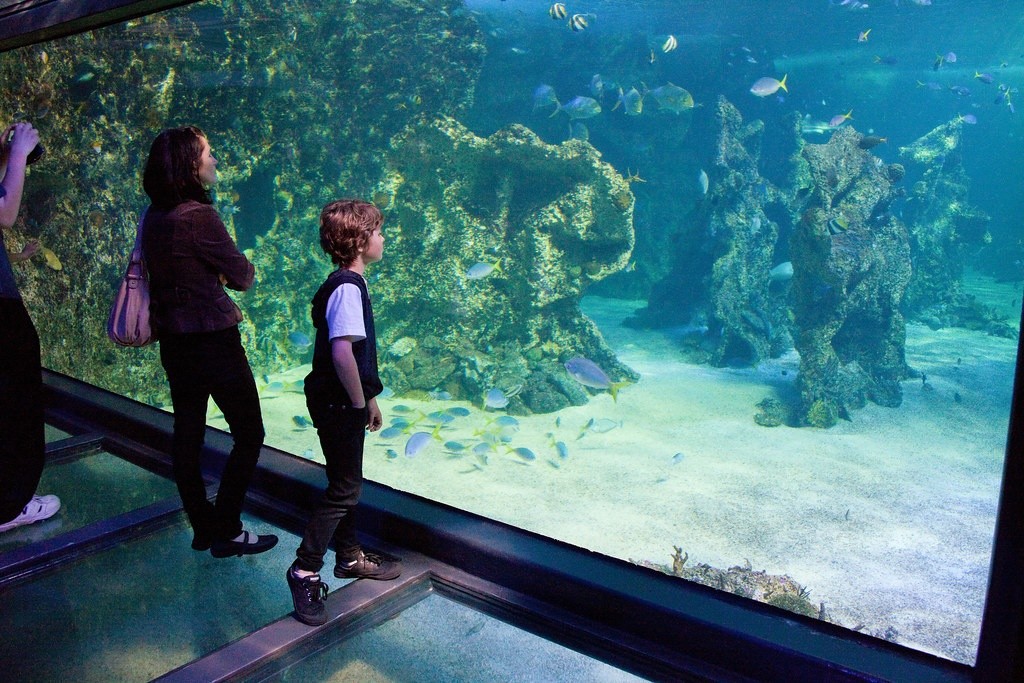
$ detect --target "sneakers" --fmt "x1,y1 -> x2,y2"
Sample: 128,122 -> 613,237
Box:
0,493 -> 61,532
285,564 -> 330,625
333,549 -> 401,580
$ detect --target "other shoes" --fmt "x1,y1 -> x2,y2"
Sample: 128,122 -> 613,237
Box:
192,528 -> 213,551
210,531 -> 278,559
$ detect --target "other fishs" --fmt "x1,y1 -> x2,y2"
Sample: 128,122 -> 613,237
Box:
38,1 -> 1023,470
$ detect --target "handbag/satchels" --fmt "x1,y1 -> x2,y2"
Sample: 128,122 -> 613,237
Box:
105,203 -> 160,348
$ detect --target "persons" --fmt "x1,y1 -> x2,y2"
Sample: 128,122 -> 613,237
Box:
0,120 -> 62,533
144,127 -> 279,558
287,199 -> 405,625
